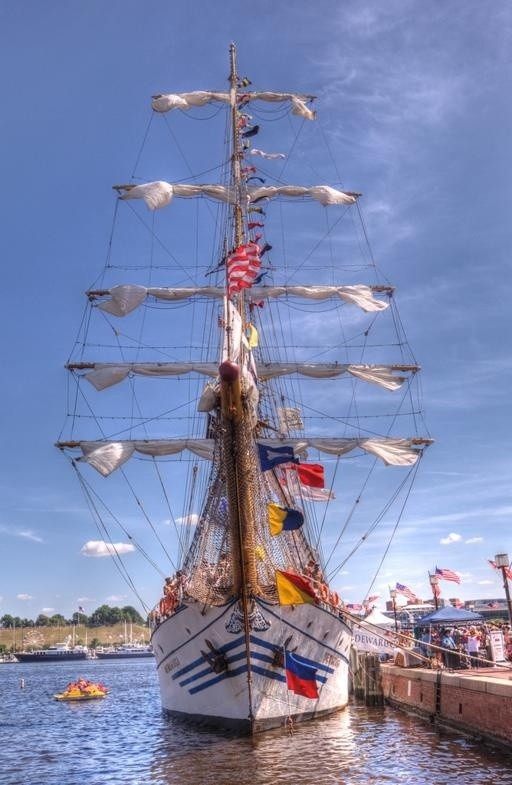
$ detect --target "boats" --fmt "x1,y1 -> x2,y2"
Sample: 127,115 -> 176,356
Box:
12,634 -> 88,662
96,643 -> 156,659
48,683 -> 110,701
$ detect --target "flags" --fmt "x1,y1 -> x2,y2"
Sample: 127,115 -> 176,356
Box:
395,583 -> 416,602
487,560 -> 496,567
364,584 -> 502,611
278,460 -> 324,488
255,442 -> 300,473
285,651 -> 320,700
266,502 -> 305,537
227,242 -> 260,297
436,568 -> 460,584
348,603 -> 362,611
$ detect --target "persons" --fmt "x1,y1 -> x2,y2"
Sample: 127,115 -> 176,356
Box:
65,677 -> 109,695
162,560 -> 347,623
384,620 -> 512,671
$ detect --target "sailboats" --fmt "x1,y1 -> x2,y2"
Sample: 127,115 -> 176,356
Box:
58,43 -> 447,739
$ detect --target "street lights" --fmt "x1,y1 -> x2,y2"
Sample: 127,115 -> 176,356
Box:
493,550 -> 512,629
427,572 -> 441,613
388,586 -> 398,639
